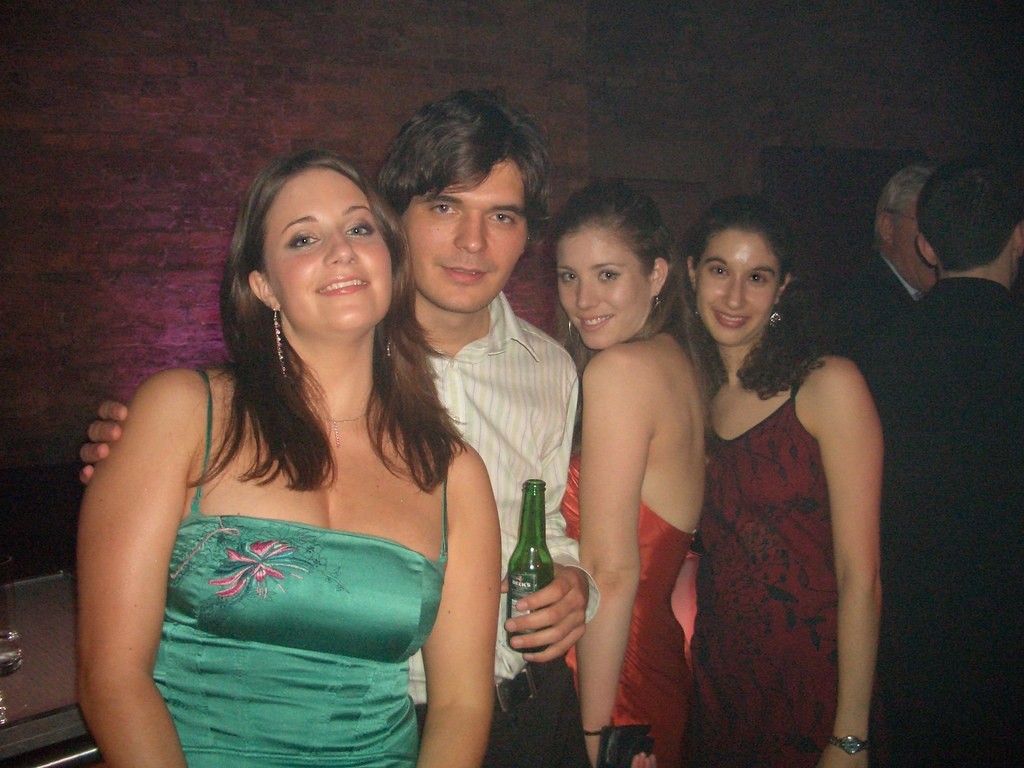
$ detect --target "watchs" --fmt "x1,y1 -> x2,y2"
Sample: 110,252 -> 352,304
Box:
828,735 -> 870,756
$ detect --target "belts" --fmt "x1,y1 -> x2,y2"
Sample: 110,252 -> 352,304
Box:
416,654 -> 569,724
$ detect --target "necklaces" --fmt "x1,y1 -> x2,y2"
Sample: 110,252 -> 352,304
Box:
317,412 -> 366,447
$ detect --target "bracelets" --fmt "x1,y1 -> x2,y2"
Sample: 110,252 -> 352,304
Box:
582,725 -> 614,736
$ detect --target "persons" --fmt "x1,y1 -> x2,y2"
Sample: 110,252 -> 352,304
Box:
80,92 -> 601,768
784,158 -> 1023,768
77,151 -> 501,768
547,181 -> 706,768
684,202 -> 883,768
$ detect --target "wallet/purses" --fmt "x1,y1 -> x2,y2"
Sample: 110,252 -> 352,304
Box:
598,724 -> 655,768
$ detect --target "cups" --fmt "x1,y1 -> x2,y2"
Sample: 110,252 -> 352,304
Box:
0,553 -> 22,678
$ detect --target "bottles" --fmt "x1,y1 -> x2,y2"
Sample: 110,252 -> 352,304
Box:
507,478 -> 550,653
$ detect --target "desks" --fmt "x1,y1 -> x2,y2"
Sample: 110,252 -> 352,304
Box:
0,567 -> 107,768
